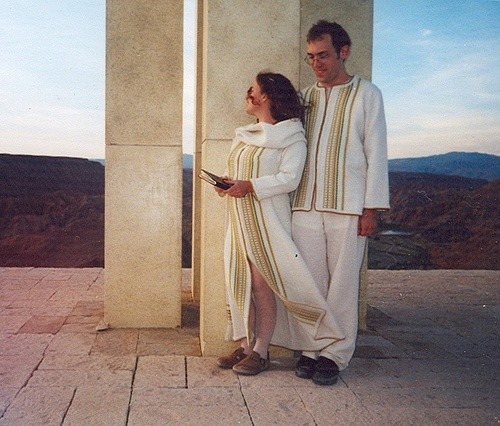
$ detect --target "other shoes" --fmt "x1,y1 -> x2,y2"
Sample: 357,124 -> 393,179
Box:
232,351 -> 272,375
215,347 -> 247,368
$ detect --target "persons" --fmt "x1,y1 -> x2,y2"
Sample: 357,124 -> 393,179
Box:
216,70 -> 343,377
295,18 -> 391,388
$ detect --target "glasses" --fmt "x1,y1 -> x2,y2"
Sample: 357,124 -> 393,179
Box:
304,51 -> 335,65
247,86 -> 255,95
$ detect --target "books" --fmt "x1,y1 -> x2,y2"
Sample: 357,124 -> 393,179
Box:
199,168 -> 231,190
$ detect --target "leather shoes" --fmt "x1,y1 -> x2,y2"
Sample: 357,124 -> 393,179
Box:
313,356 -> 339,386
295,356 -> 316,378
